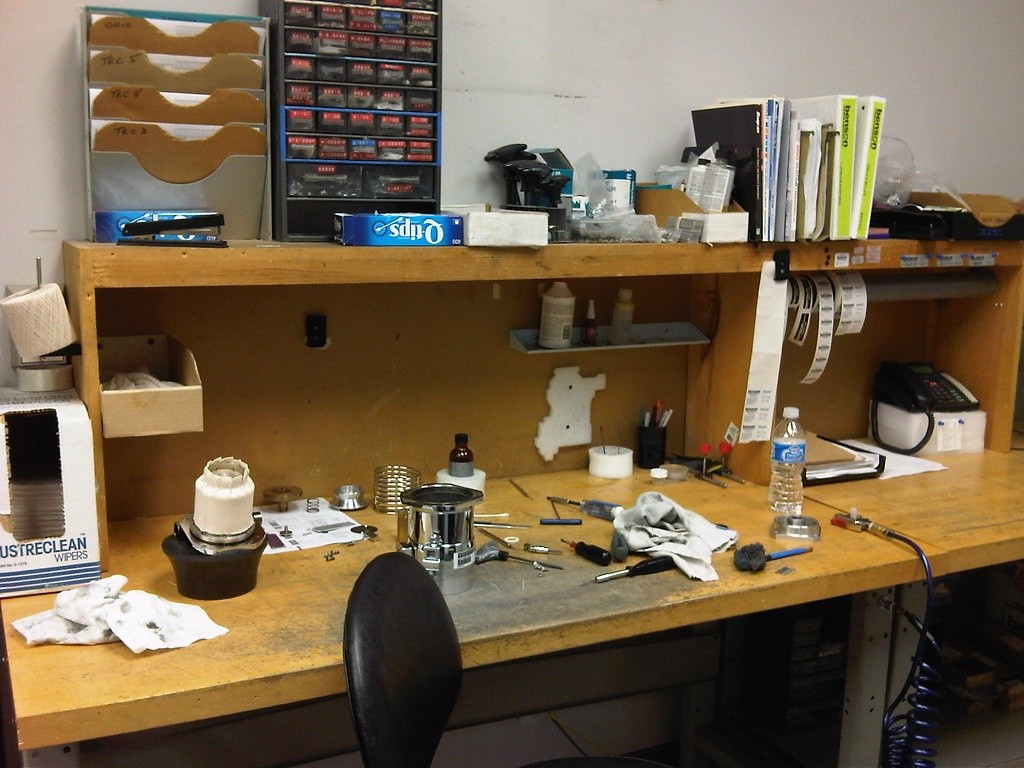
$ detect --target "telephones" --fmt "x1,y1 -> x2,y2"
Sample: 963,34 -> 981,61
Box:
871,360 -> 981,414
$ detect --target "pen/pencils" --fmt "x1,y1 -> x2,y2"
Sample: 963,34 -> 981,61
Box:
765,546 -> 813,561
638,396 -> 675,427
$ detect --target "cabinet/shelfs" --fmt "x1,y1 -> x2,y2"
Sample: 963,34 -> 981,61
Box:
255,0 -> 444,238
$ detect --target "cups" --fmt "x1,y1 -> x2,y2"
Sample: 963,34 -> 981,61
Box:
638,425 -> 667,469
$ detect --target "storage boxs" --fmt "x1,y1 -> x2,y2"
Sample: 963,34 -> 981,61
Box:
634,182 -> 749,244
571,194 -> 590,219
908,191 -> 1018,227
0,384 -> 101,601
440,203 -> 549,247
868,206 -> 1024,239
334,212 -> 464,246
868,395 -> 986,453
97,334 -> 204,439
520,146 -> 574,219
286,163 -> 434,201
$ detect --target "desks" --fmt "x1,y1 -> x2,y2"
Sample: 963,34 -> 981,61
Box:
779,239 -> 1024,768
0,235 -> 948,768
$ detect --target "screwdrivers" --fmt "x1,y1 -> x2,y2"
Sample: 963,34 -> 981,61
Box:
546,495 -> 621,521
559,537 -> 611,566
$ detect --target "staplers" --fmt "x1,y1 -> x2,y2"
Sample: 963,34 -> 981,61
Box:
115,212 -> 231,248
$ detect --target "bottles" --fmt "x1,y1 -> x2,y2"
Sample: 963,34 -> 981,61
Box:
608,287 -> 637,344
768,406 -> 806,516
448,433 -> 474,478
538,278 -> 576,349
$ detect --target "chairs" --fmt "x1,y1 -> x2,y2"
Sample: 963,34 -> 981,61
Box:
342,552 -> 669,768
